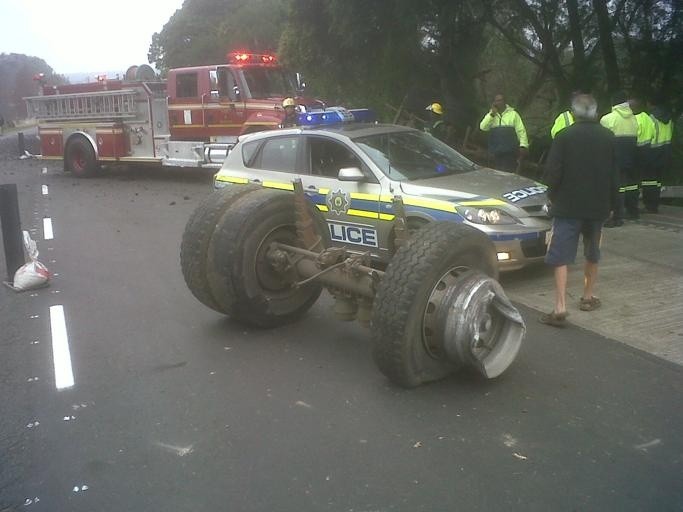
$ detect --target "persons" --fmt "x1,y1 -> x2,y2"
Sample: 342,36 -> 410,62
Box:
422,101 -> 448,144
276,95 -> 300,129
477,92 -> 533,175
548,86 -> 676,230
536,91 -> 624,331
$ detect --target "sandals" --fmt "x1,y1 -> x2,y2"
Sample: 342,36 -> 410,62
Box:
579,296 -> 601,311
539,310 -> 569,326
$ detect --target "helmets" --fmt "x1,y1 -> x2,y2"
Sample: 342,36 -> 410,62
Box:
426,103 -> 443,115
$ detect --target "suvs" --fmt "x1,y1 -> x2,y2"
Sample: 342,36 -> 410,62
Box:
211,100 -> 555,277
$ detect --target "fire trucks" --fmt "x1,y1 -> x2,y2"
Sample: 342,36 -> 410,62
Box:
20,49 -> 326,180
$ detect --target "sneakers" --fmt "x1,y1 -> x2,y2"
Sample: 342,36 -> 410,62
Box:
604,205 -> 657,227
